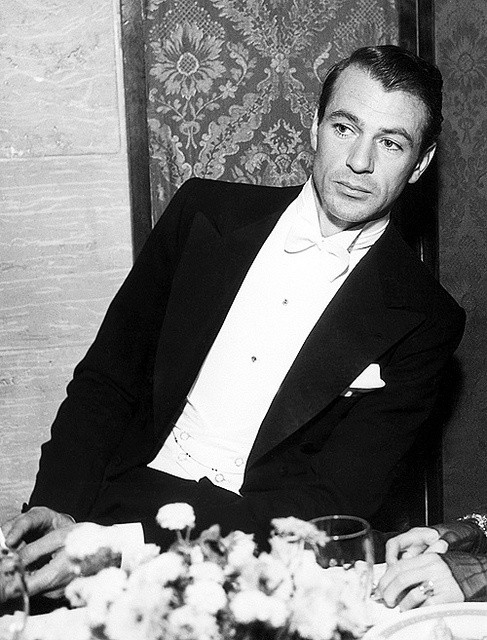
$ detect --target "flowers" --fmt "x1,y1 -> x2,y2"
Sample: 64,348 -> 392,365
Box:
65,503 -> 374,640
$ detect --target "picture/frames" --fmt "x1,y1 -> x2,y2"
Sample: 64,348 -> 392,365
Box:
118,0 -> 440,287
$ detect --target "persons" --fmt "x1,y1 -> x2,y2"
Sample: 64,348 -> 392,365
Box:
0,46 -> 467,617
372,501 -> 487,613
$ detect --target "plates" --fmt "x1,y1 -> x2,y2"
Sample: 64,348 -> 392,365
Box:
358,602 -> 487,640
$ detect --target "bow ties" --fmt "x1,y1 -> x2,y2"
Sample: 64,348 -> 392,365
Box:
282,218 -> 349,282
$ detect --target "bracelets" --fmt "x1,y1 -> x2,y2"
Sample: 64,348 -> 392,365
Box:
456,511 -> 487,537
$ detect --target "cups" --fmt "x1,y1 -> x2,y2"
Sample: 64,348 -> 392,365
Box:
305,516 -> 375,606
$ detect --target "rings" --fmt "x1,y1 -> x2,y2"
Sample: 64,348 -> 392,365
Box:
420,581 -> 434,597
